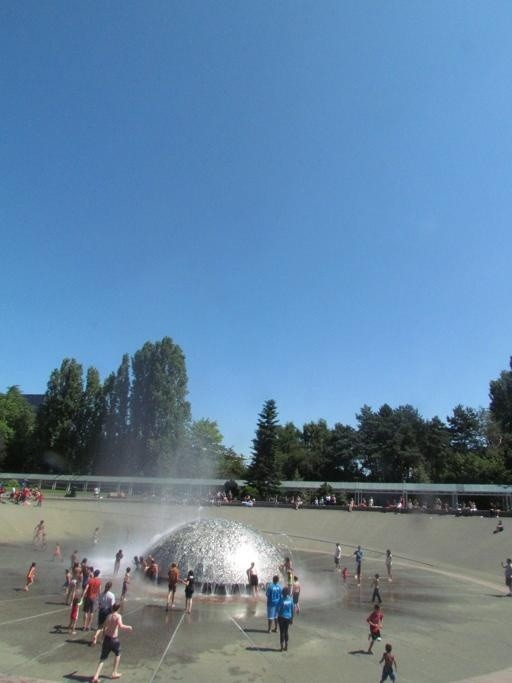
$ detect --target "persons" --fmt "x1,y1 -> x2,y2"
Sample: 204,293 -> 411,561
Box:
386,549 -> 394,582
92,527 -> 102,547
244,495 -> 251,502
286,569 -> 292,588
370,572 -> 382,604
81,557 -> 89,587
82,570 -> 102,631
94,487 -> 99,498
283,495 -> 302,510
62,568 -> 72,595
278,588 -> 295,653
86,565 -> 94,580
362,496 -> 373,506
180,570 -> 196,614
353,544 -> 364,578
113,549 -> 123,578
52,542 -> 63,563
68,598 -> 83,634
292,576 -> 301,614
265,575 -> 281,633
501,558 -> 512,597
205,489 -> 232,503
120,567 -> 133,602
41,533 -> 47,551
91,581 -> 116,647
133,555 -> 159,586
383,496 -> 403,512
72,561 -> 84,589
349,498 -> 354,506
246,562 -> 259,597
495,520 -> 504,534
64,486 -> 76,497
342,567 -> 348,582
281,558 -> 292,573
92,603 -> 133,683
72,548 -> 80,568
366,604 -> 384,653
9,485 -> 43,508
315,494 -> 336,505
490,501 -> 501,512
379,644 -> 398,683
166,562 -> 180,609
408,497 -> 475,511
33,520 -> 45,546
333,542 -> 342,568
24,561 -> 36,592
